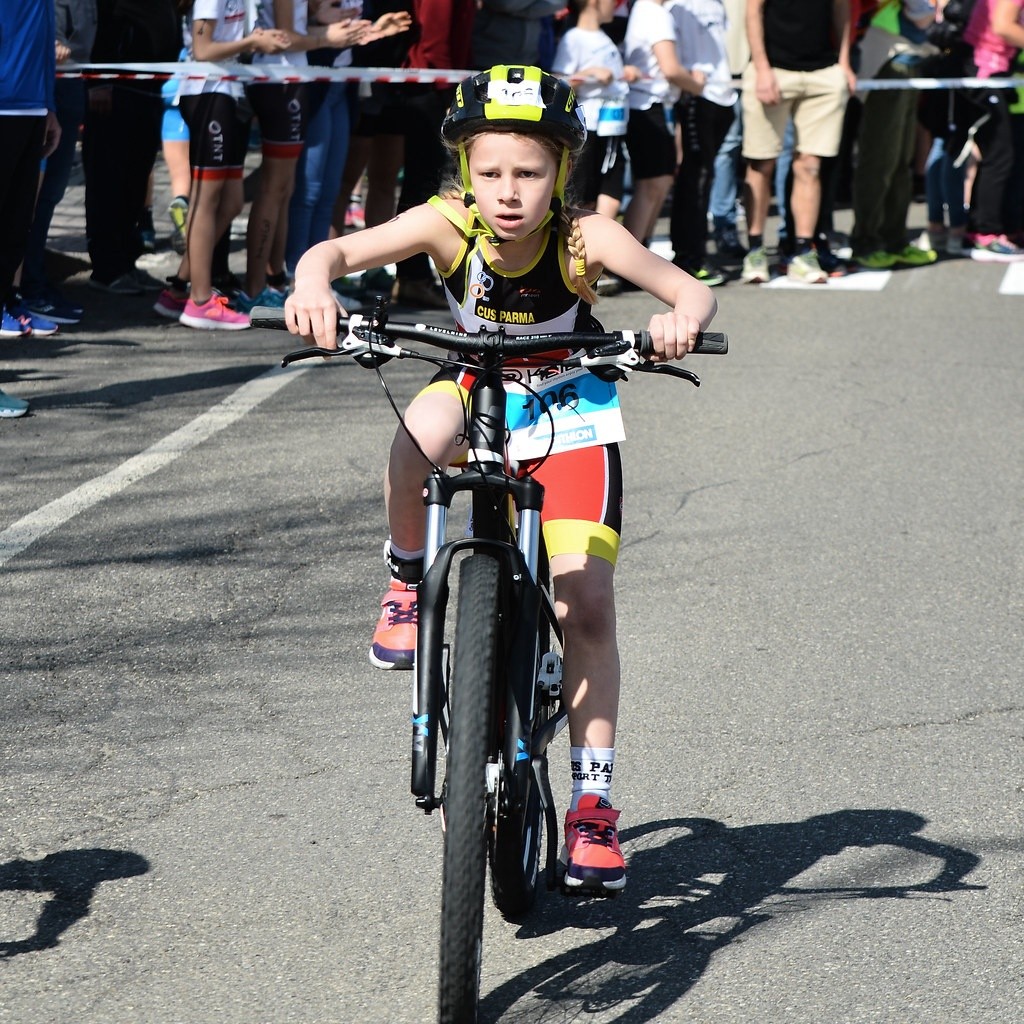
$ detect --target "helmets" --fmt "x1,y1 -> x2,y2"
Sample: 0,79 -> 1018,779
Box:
441,66 -> 587,149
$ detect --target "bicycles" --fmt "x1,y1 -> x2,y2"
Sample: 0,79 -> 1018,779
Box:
247,306 -> 728,1024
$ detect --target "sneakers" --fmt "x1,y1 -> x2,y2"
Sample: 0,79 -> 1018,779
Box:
563,795 -> 626,889
1,389 -> 29,418
369,581 -> 418,669
673,204 -> 1024,287
1,196 -> 456,336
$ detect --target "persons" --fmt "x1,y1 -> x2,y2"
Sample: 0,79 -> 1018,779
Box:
0,0 -> 1024,336
282,64 -> 718,891
